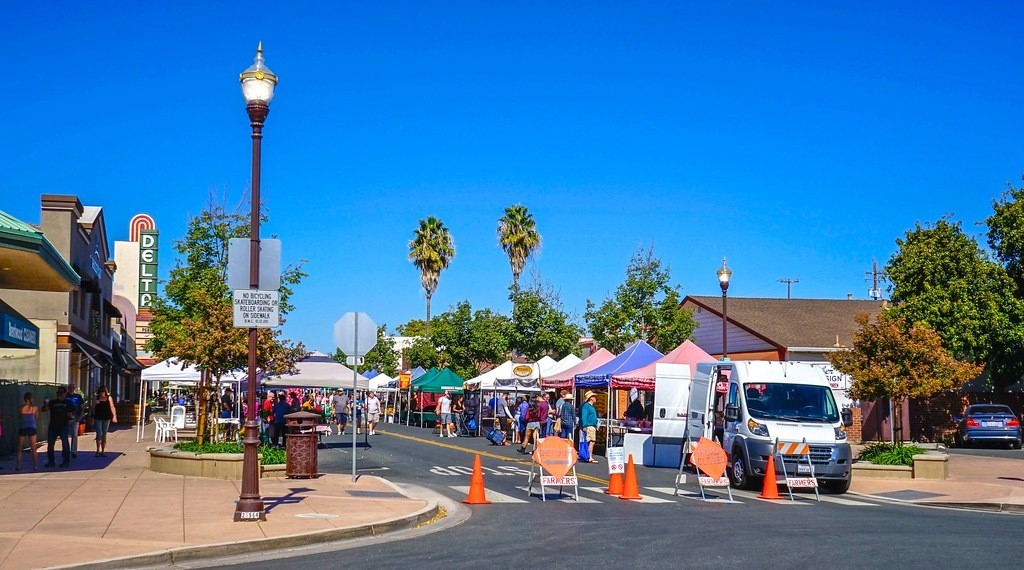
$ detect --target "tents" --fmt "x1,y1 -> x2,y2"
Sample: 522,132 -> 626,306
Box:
361,339 -> 731,457
238,350 -> 369,450
136,355 -> 249,444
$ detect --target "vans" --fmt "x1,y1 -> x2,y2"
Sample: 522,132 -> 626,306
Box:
651,360 -> 854,494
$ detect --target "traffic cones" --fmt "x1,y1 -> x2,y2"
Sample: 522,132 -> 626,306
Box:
462,454 -> 493,505
604,454 -> 644,500
755,453 -> 785,501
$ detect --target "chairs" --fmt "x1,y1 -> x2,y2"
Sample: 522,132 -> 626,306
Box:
154,416 -> 177,443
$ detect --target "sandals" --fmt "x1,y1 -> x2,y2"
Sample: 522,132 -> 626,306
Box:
586,458 -> 598,464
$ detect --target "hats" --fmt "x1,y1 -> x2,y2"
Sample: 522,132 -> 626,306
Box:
562,394 -> 574,399
338,388 -> 343,391
503,392 -> 510,395
585,390 -> 597,402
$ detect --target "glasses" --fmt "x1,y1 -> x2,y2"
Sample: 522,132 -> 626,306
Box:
56,392 -> 62,394
98,391 -> 105,393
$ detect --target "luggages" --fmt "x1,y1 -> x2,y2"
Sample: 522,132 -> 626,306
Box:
486,420 -> 515,445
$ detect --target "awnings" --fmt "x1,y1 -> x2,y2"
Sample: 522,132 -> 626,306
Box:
69,340 -> 144,378
103,298 -> 122,318
73,265 -> 96,291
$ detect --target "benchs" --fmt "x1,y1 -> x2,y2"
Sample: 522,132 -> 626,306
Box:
912,453 -> 950,479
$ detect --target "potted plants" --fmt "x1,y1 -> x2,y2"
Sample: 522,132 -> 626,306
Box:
73,387 -> 90,436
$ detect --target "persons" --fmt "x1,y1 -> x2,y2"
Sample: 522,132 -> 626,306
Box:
243,391 -> 300,450
435,390 -> 654,464
154,389 -> 185,414
91,385 -> 117,458
12,392 -> 39,471
301,388 -> 417,436
41,383 -> 84,469
221,388 -> 238,442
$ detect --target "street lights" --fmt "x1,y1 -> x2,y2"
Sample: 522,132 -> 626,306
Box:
230,36 -> 283,523
714,258 -> 733,360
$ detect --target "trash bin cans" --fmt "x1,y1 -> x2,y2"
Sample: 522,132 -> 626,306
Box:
283,411 -> 321,480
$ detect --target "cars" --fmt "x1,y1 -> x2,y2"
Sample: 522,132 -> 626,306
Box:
953,403 -> 1022,450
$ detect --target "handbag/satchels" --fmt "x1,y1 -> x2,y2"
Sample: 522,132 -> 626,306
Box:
578,431 -> 590,461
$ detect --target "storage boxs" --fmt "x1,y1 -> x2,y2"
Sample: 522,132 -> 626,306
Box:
487,429 -> 506,445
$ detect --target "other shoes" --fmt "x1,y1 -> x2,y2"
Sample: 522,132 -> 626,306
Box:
59,464 -> 70,468
45,463 -> 55,467
440,433 -> 443,437
369,429 -> 375,435
337,431 -> 345,435
95,453 -> 107,457
529,450 -> 534,454
448,434 -> 454,438
517,446 -> 526,454
452,432 -> 457,437
